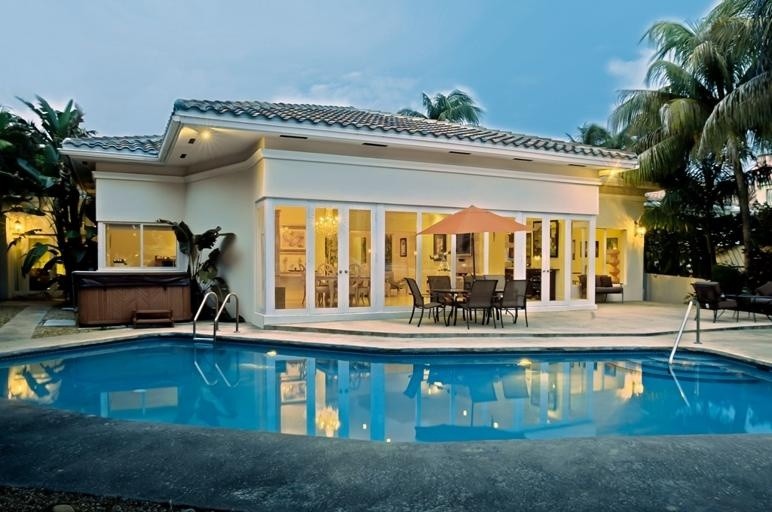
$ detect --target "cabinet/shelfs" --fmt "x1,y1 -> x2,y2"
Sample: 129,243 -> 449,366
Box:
505,268 -> 560,301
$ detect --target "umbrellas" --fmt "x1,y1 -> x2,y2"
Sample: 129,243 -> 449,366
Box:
416,204 -> 539,279
409,392 -> 528,444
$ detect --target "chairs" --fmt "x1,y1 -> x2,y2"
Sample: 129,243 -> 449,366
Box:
299,262 -> 391,308
404,274 -> 532,331
577,274 -> 623,303
690,280 -> 772,323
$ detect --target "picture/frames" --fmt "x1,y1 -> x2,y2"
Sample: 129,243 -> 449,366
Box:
533,219 -> 559,257
400,237 -> 407,257
433,234 -> 446,261
